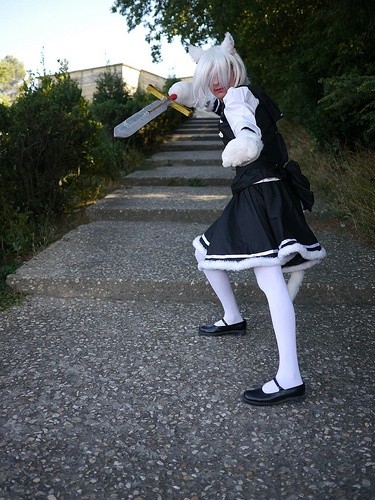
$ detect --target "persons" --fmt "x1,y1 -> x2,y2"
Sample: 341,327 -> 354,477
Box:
169,31 -> 325,407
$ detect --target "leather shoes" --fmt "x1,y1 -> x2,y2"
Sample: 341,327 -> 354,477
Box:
244,377 -> 305,406
198,318 -> 247,337
